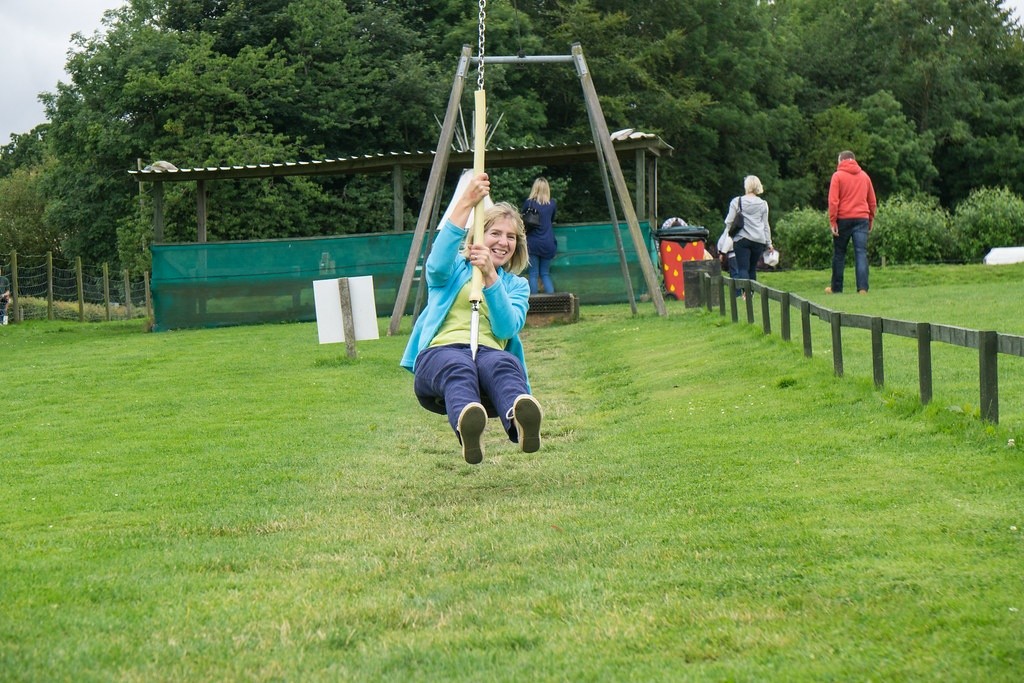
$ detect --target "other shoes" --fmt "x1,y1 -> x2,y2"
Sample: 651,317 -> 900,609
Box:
506,393 -> 542,454
458,402 -> 488,465
858,288 -> 866,295
741,291 -> 746,300
825,287 -> 834,294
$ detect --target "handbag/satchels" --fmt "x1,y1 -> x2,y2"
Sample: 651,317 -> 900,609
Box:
523,198 -> 540,233
729,196 -> 743,238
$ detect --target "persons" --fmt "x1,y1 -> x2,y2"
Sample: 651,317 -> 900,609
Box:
717,175 -> 774,301
0,267 -> 10,323
520,176 -> 558,294
398,173 -> 543,465
825,150 -> 877,294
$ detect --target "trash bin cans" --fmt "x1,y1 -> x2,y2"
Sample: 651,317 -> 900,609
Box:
658,225 -> 710,301
